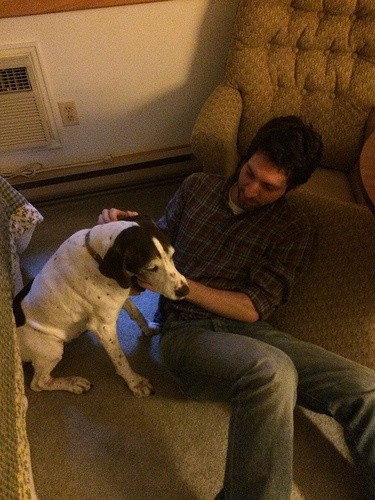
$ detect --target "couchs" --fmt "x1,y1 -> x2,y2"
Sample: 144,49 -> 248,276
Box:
188,1 -> 375,214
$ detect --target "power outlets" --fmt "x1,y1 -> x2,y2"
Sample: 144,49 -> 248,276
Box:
58,101 -> 80,128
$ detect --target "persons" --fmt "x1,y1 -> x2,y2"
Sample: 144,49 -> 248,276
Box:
97,115 -> 375,500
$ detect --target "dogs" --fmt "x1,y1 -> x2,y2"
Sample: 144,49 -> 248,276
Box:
12,214 -> 190,399
